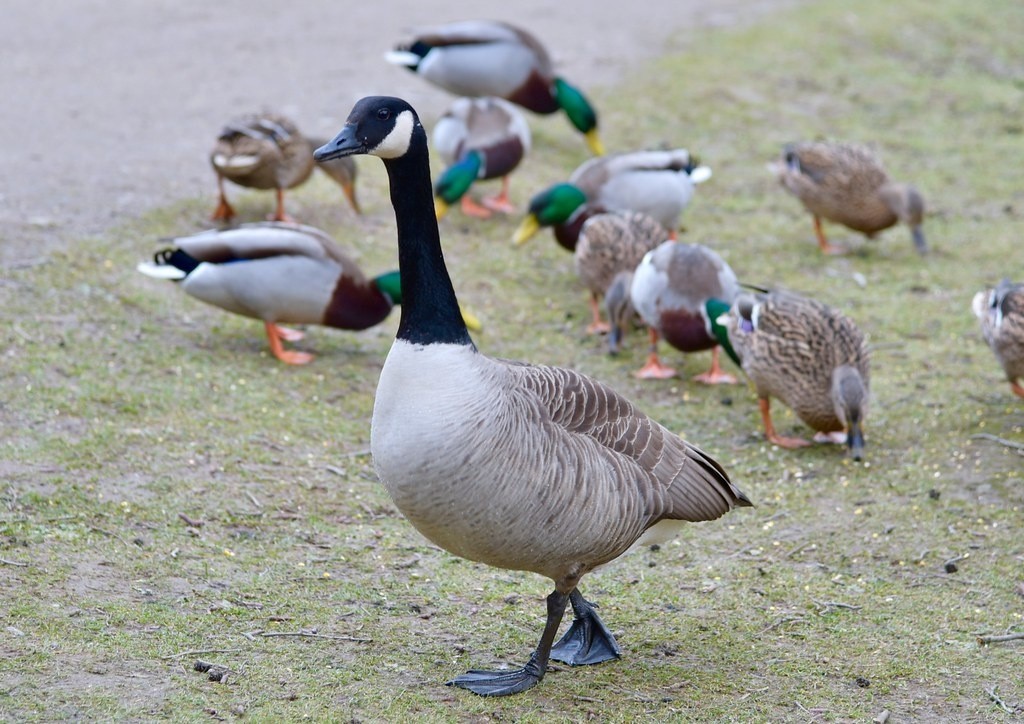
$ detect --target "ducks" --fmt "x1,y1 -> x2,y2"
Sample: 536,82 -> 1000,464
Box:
139,221 -> 482,371
505,147 -> 714,259
764,134 -> 933,261
697,279 -> 874,465
385,18 -> 613,157
432,97 -> 533,228
312,96 -> 759,703
204,110 -> 369,223
969,271 -> 1023,401
566,196 -> 669,337
603,239 -> 742,389
